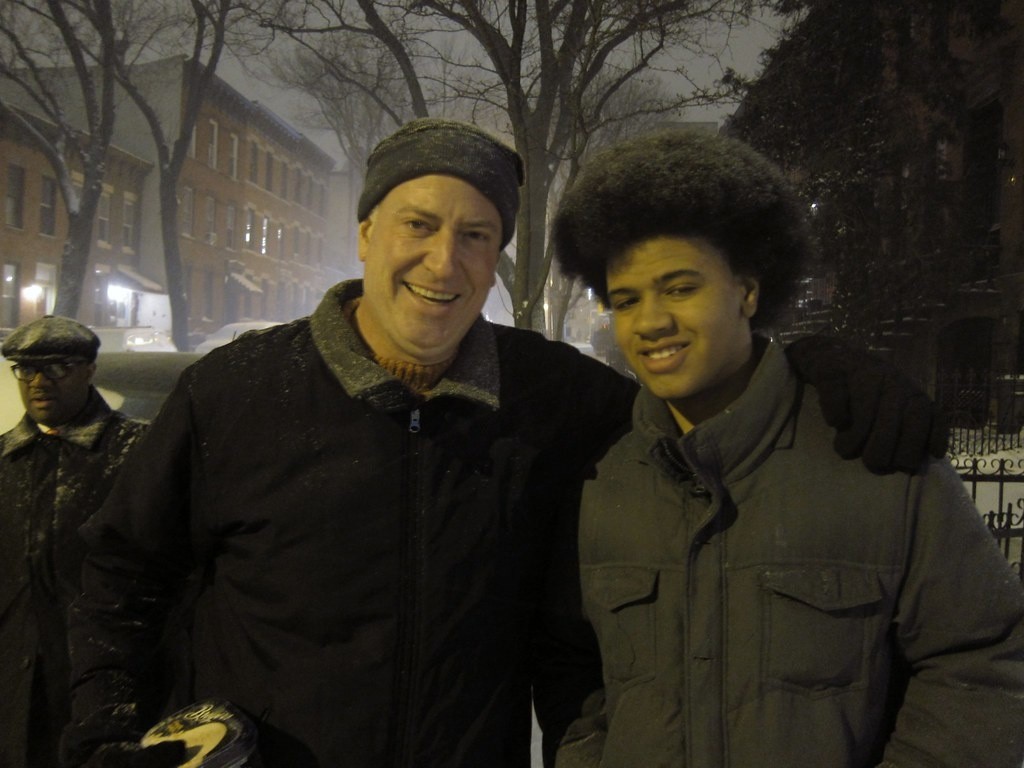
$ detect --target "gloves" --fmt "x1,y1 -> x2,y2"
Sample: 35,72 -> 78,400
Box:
59,707 -> 187,768
785,336 -> 934,474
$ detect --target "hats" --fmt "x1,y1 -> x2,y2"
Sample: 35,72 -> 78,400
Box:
1,314 -> 101,367
356,119 -> 524,255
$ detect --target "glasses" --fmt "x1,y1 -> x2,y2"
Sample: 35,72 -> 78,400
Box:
10,359 -> 85,382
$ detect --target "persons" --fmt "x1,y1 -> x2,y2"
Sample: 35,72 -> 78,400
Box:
552,130 -> 1024,768
65,115 -> 951,768
0,315 -> 150,768
